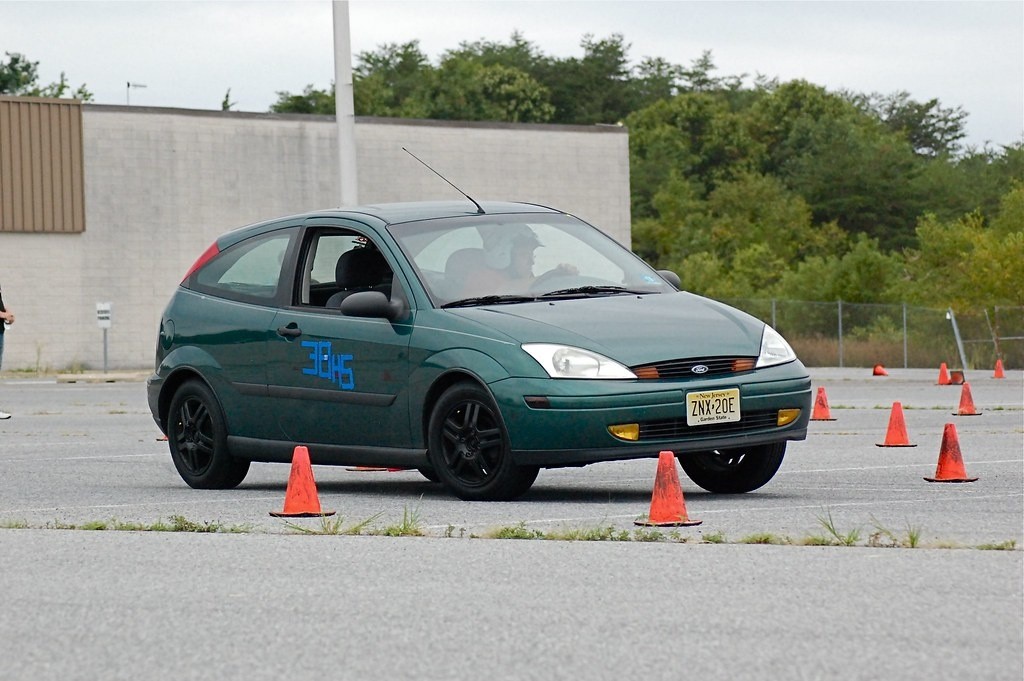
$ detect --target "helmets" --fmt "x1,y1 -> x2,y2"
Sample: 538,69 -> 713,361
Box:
483,222 -> 546,269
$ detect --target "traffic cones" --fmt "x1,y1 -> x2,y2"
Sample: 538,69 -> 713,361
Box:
950,369 -> 965,385
922,423 -> 979,483
952,381 -> 983,416
934,362 -> 952,386
991,359 -> 1007,379
875,401 -> 917,447
634,450 -> 703,527
269,446 -> 336,518
873,362 -> 889,377
808,385 -> 837,421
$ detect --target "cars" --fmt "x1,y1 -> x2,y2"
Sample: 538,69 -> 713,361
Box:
146,198 -> 812,502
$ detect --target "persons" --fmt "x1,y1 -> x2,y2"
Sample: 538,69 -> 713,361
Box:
0,284 -> 15,419
463,222 -> 579,297
